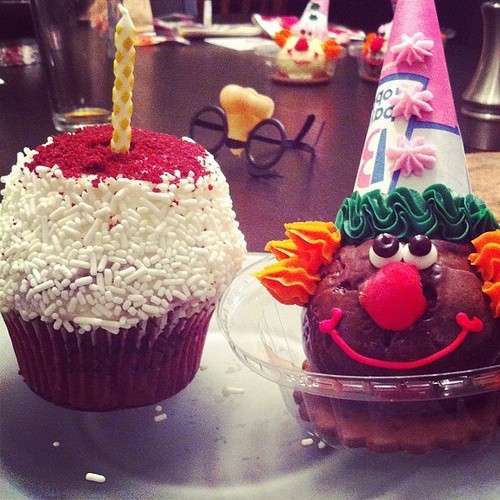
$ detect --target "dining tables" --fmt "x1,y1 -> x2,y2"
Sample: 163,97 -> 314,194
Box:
0,16 -> 500,499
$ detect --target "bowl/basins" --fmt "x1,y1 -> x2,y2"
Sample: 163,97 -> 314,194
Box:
219,257 -> 500,458
348,43 -> 388,82
254,43 -> 345,84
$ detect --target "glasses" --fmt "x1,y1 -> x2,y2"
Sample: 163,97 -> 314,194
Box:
187,105 -> 320,172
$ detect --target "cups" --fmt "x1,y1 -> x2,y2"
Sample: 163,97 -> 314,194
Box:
30,0 -> 122,133
